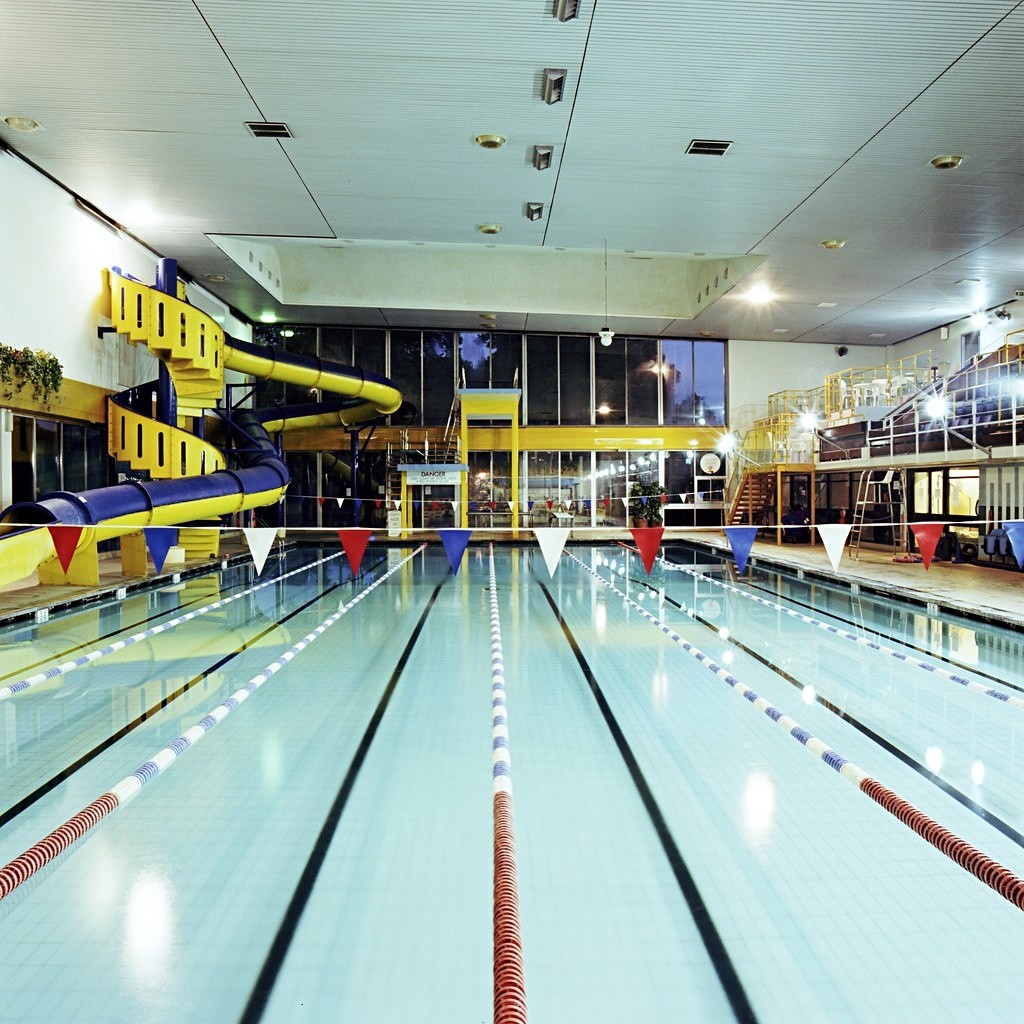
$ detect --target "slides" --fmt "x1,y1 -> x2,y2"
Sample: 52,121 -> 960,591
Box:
0,322 -> 400,590
